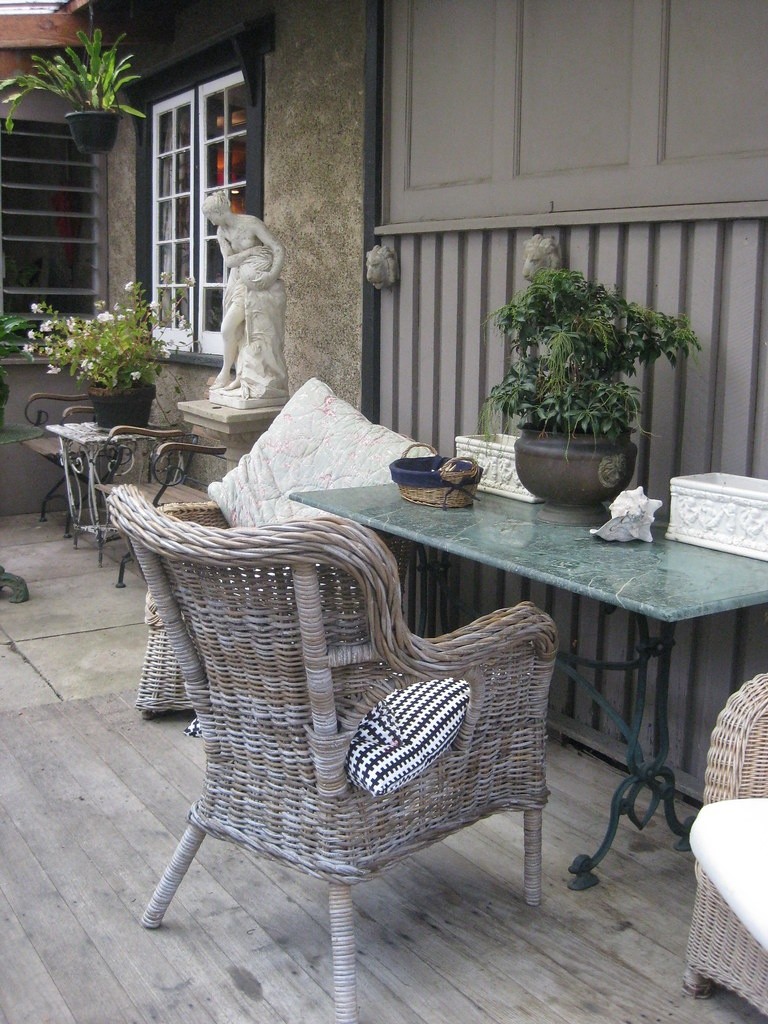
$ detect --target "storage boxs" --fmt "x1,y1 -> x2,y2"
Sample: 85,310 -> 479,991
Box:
455,432 -> 545,503
665,471 -> 768,563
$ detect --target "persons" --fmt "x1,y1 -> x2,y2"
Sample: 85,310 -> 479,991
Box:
202,189 -> 285,391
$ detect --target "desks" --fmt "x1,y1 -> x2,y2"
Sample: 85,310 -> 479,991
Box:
45,422 -> 183,568
289,483 -> 768,891
0,423 -> 45,603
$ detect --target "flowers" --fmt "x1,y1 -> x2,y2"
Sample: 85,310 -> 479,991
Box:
21,270 -> 202,388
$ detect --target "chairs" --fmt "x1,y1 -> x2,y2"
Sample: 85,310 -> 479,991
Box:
20,378 -> 118,538
105,482 -> 558,1023
682,672 -> 768,1017
95,377 -> 234,587
136,433 -> 434,719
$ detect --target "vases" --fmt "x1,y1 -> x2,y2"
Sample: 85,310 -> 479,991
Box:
87,383 -> 156,433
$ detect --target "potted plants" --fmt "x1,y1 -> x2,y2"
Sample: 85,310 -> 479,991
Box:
0,28 -> 146,154
475,268 -> 702,526
0,314 -> 36,432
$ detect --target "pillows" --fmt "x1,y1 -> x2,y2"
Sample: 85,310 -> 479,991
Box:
690,798 -> 768,951
182,669 -> 503,798
208,377 -> 434,528
